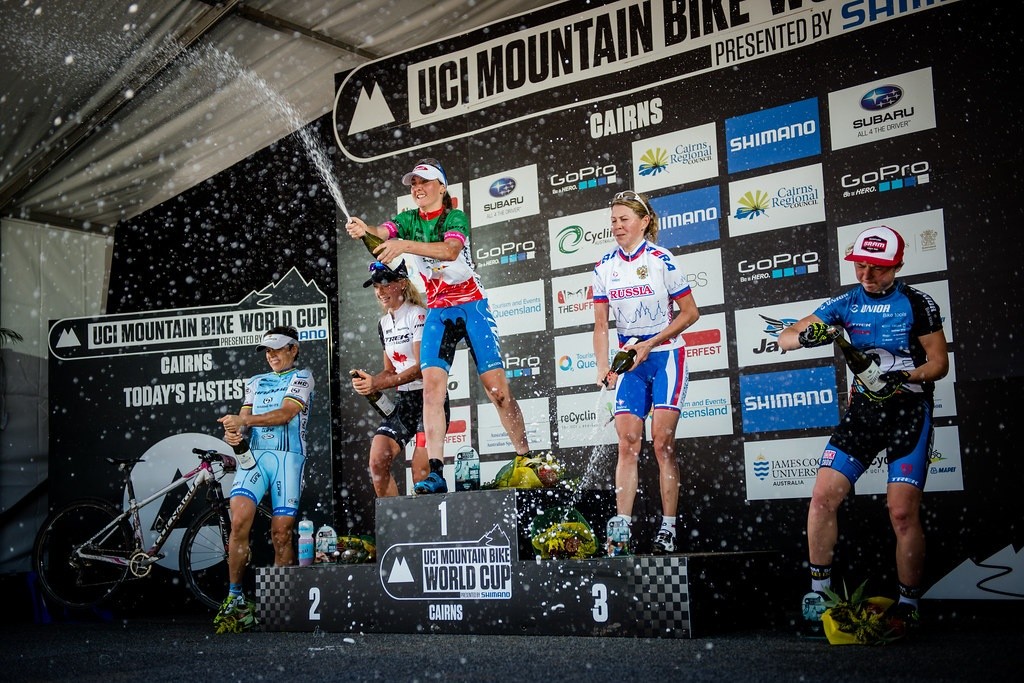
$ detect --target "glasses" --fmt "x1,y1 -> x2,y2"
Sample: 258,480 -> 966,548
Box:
615,190 -> 651,215
370,262 -> 388,272
414,157 -> 447,181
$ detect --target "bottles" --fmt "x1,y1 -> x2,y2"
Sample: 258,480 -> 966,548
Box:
298,518 -> 315,566
229,430 -> 257,470
349,370 -> 398,419
351,220 -> 405,272
828,326 -> 892,397
602,336 -> 644,387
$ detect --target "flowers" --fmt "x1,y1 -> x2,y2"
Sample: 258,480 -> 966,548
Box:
335,536 -> 377,564
215,600 -> 255,636
486,451 -> 581,497
531,522 -> 601,562
816,578 -> 902,648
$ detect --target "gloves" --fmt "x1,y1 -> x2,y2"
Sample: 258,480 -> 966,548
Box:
798,322 -> 841,348
863,370 -> 911,403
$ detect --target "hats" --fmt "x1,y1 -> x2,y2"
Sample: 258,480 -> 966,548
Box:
255,333 -> 299,352
401,164 -> 448,188
362,258 -> 409,288
844,225 -> 905,267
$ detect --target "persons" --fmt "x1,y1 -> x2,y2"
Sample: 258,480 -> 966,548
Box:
214,325 -> 315,632
777,226 -> 949,643
592,189 -> 700,554
351,258 -> 450,497
344,158 -> 542,495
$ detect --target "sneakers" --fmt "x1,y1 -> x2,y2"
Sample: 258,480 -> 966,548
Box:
413,471 -> 448,494
650,522 -> 680,555
212,593 -> 246,624
604,515 -> 633,556
868,600 -> 920,643
795,593 -> 844,640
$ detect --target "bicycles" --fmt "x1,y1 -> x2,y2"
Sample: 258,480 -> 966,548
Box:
31,448 -> 299,613
